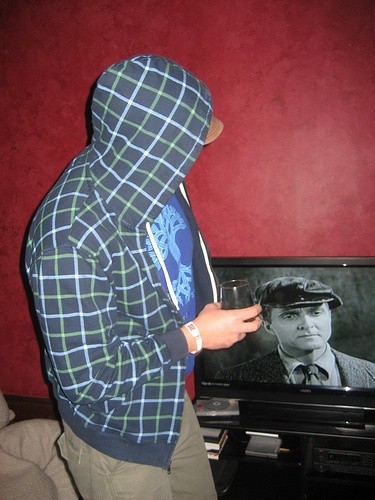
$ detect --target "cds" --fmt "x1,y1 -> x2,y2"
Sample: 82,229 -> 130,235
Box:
204,399 -> 230,410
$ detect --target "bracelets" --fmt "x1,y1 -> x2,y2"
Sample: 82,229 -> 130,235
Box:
184,321 -> 202,355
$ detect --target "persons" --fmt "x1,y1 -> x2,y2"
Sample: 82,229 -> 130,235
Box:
25,55 -> 263,500
216,275 -> 375,389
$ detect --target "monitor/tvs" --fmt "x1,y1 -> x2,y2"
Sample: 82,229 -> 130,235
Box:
194,256 -> 375,429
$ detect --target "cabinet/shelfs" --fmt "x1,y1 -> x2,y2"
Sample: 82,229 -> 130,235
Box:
191,398 -> 375,500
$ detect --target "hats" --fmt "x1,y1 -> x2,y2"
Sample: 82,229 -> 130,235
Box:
252,277 -> 344,310
205,115 -> 224,144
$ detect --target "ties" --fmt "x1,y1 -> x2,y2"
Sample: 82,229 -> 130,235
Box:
304,365 -> 323,385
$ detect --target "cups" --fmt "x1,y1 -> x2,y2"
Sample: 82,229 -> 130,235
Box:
220,279 -> 255,323
